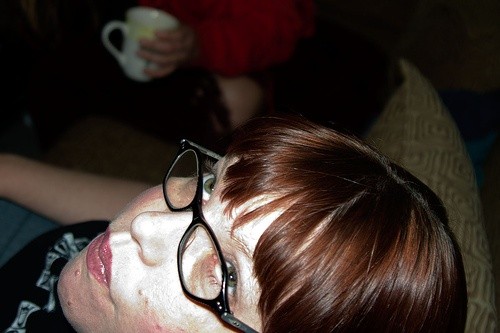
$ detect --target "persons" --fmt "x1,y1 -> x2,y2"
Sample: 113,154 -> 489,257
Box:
138,0 -> 317,77
1,127 -> 470,333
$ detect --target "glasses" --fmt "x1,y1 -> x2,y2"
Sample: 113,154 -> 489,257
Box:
163,140 -> 259,333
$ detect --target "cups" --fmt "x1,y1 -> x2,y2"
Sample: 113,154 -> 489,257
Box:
101,7 -> 179,83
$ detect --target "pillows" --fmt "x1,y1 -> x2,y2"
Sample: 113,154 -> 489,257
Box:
356,57 -> 497,333
